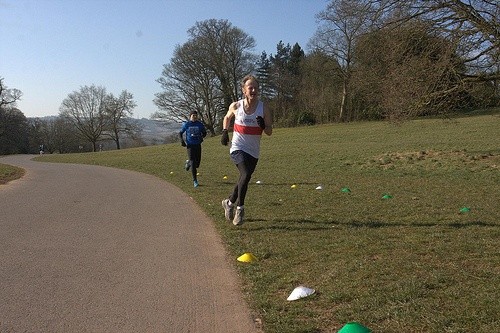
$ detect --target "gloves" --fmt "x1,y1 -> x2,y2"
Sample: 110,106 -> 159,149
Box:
221,128 -> 230,147
180,139 -> 187,148
255,115 -> 265,129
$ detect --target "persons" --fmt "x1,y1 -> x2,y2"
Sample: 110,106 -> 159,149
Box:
220,75 -> 272,225
179,111 -> 207,188
40,150 -> 44,157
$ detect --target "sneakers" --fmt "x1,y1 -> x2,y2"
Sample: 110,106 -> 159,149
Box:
193,180 -> 199,188
233,206 -> 245,225
222,198 -> 233,221
185,160 -> 190,171
